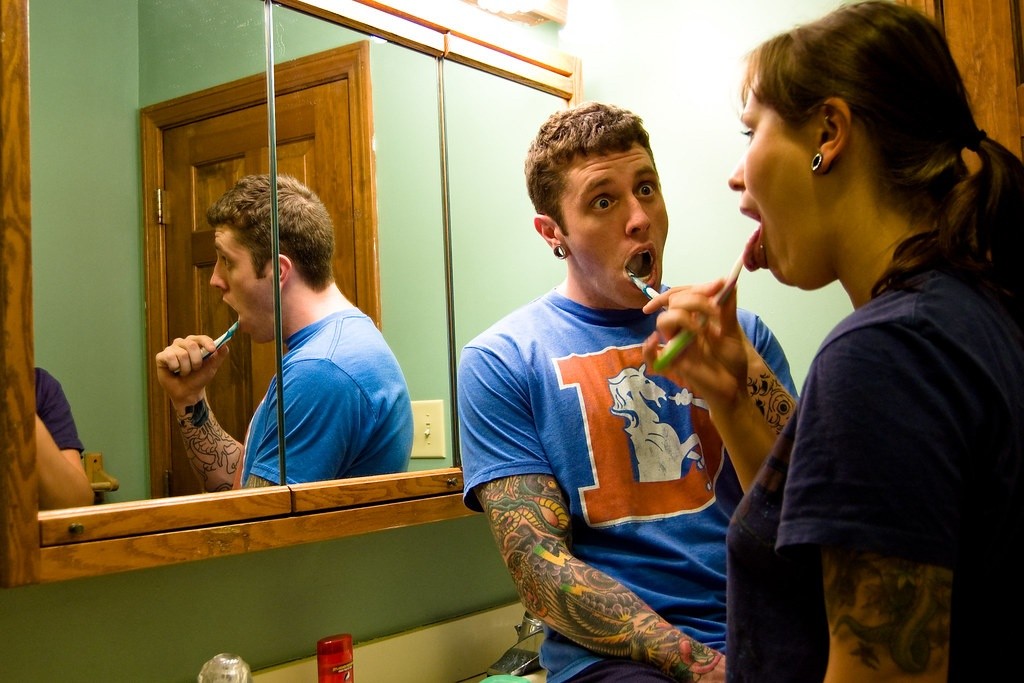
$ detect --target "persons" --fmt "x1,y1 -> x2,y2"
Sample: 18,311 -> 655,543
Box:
149,171 -> 416,496
720,1 -> 1024,683
33,364 -> 97,512
454,100 -> 802,683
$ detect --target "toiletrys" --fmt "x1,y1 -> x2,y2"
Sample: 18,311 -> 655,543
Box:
316,632 -> 354,683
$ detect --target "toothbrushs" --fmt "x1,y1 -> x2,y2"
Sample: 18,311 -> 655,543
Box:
652,248 -> 744,372
624,263 -> 672,313
173,320 -> 239,377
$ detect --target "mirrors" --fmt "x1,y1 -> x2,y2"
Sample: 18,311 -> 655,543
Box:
0,2 -> 586,581
